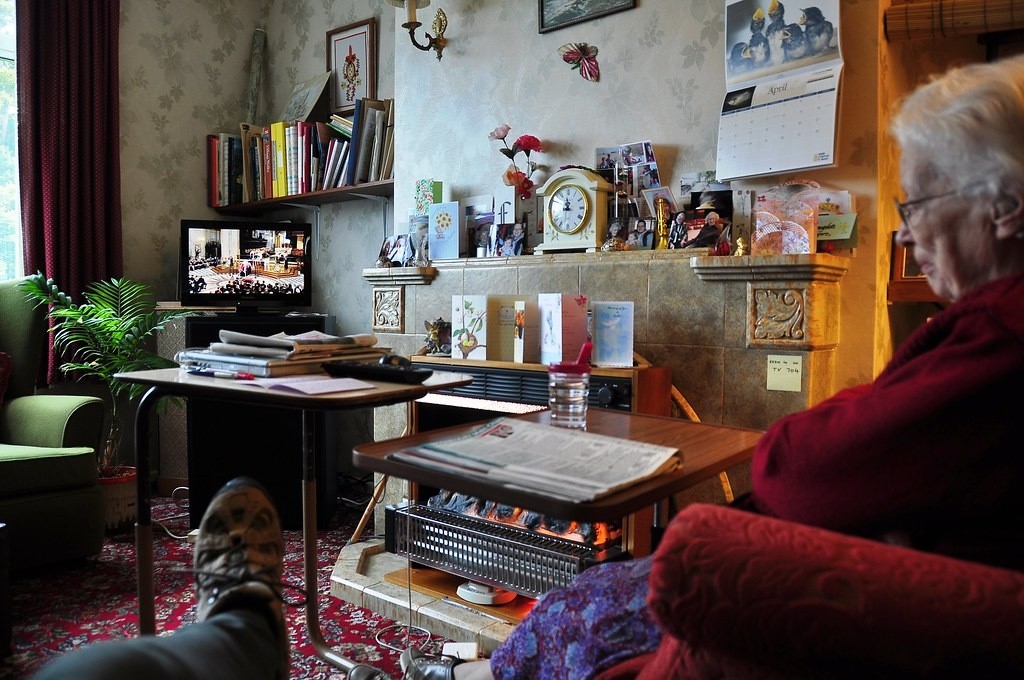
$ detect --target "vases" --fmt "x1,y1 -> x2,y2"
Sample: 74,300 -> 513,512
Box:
519,194 -> 528,254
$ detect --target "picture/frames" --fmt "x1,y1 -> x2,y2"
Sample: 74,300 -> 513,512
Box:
459,193 -> 494,258
537,0 -> 637,35
514,185 -> 544,256
325,16 -> 378,119
889,229 -> 932,282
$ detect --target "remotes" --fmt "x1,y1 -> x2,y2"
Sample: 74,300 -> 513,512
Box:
321,359 -> 433,386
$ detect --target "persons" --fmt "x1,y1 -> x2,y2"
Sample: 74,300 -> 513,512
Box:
607,222 -> 621,239
189,251 -> 305,294
498,223 -> 524,257
685,212 -> 721,248
383,237 -> 404,257
32,479 -> 291,680
668,213 -> 688,249
600,153 -> 615,168
622,148 -> 643,166
347,56 -> 1024,680
653,194 -> 674,228
647,143 -> 653,162
625,220 -> 653,248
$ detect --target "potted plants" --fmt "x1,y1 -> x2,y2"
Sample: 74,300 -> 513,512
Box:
20,270 -> 209,534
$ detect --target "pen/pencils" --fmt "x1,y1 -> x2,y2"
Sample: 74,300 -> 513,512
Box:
187,370 -> 237,378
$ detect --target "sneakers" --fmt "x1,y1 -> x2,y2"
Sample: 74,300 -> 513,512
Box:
192,480 -> 309,680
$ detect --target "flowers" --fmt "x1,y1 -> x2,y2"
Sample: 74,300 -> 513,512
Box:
487,125 -> 543,197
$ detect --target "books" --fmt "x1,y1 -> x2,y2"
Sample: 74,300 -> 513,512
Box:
236,373 -> 377,395
451,293 -> 634,368
207,98 -> 394,206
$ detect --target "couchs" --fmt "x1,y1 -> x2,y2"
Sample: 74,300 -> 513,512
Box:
594,500 -> 1024,680
0,275 -> 106,578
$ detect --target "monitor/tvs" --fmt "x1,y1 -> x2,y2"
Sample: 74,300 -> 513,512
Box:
178,219 -> 312,315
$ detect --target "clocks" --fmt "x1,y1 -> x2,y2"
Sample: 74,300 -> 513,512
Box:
533,167 -> 613,257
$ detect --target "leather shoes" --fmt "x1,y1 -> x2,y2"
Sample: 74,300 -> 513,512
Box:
345,664 -> 393,680
400,646 -> 469,680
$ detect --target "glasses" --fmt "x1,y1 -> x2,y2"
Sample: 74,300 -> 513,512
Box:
892,180 -> 987,228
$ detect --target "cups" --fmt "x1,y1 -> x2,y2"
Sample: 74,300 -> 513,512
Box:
547,360 -> 593,432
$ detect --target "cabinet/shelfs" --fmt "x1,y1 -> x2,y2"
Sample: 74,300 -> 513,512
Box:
156,314 -> 328,534
410,353 -> 673,531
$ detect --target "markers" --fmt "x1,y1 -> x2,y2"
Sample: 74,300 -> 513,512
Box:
196,367 -> 254,380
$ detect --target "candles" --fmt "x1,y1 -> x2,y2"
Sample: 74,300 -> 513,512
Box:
404,0 -> 417,24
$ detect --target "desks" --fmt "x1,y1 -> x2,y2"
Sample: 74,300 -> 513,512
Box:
114,367 -> 474,676
345,406 -> 768,562
